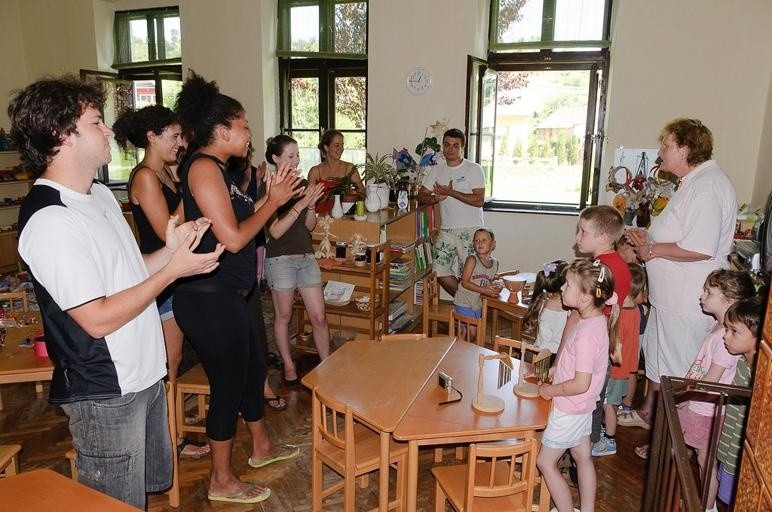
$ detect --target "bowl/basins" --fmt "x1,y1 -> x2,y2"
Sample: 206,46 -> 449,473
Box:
503,277 -> 527,292
33,333 -> 48,357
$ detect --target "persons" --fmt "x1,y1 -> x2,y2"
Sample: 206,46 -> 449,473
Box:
259,134 -> 330,391
575,203 -> 630,458
225,142 -> 288,411
454,227 -> 502,343
306,130 -> 365,216
113,104 -> 210,459
623,116 -> 739,459
535,258 -> 610,512
168,66 -> 301,503
7,70 -> 226,510
617,237 -> 645,410
522,259 -> 573,362
670,267 -> 757,499
590,262 -> 646,459
716,300 -> 762,510
560,361 -> 612,489
417,128 -> 486,299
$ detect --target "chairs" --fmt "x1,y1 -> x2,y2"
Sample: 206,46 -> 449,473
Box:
309,384 -> 408,512
447,309 -> 483,346
0,443 -> 24,477
422,271 -> 457,340
430,438 -> 536,512
493,334 -> 525,359
0,290 -> 29,311
63,380 -> 181,509
174,360 -> 216,439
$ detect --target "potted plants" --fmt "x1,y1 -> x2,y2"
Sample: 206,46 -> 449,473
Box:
357,151 -> 396,209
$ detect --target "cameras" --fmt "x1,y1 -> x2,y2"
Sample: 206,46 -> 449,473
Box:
438,370 -> 453,394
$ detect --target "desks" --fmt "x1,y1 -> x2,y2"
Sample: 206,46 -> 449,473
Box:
300,336 -> 456,512
0,467 -> 138,512
1,311 -> 62,385
478,270 -> 541,350
392,336 -> 552,512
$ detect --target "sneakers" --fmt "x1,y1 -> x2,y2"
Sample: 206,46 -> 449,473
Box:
590,430 -> 620,458
556,453 -> 578,488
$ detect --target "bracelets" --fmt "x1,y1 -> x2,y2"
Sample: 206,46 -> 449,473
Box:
288,207 -> 300,221
646,239 -> 656,260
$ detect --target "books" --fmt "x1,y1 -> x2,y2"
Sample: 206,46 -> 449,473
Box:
391,314 -> 412,330
416,210 -> 429,241
415,244 -> 434,273
415,281 -> 434,306
388,299 -> 406,322
386,237 -> 416,255
389,258 -> 414,291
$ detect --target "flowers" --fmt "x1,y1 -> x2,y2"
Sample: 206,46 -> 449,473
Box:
329,164 -> 361,193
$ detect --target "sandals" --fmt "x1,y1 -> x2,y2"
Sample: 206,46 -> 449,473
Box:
283,374 -> 301,389
614,406 -> 651,430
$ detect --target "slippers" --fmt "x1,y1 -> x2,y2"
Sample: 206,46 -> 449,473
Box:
249,443 -> 300,468
174,438 -> 212,459
634,444 -> 657,461
266,397 -> 291,414
205,480 -> 271,502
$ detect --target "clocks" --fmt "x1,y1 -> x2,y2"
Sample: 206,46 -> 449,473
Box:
406,66 -> 432,96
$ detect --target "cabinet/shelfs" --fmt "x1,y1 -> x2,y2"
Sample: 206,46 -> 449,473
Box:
288,239 -> 393,349
0,136 -> 30,275
294,196 -> 438,360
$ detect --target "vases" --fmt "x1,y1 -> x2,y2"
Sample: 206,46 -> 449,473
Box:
331,194 -> 343,219
364,183 -> 380,213
